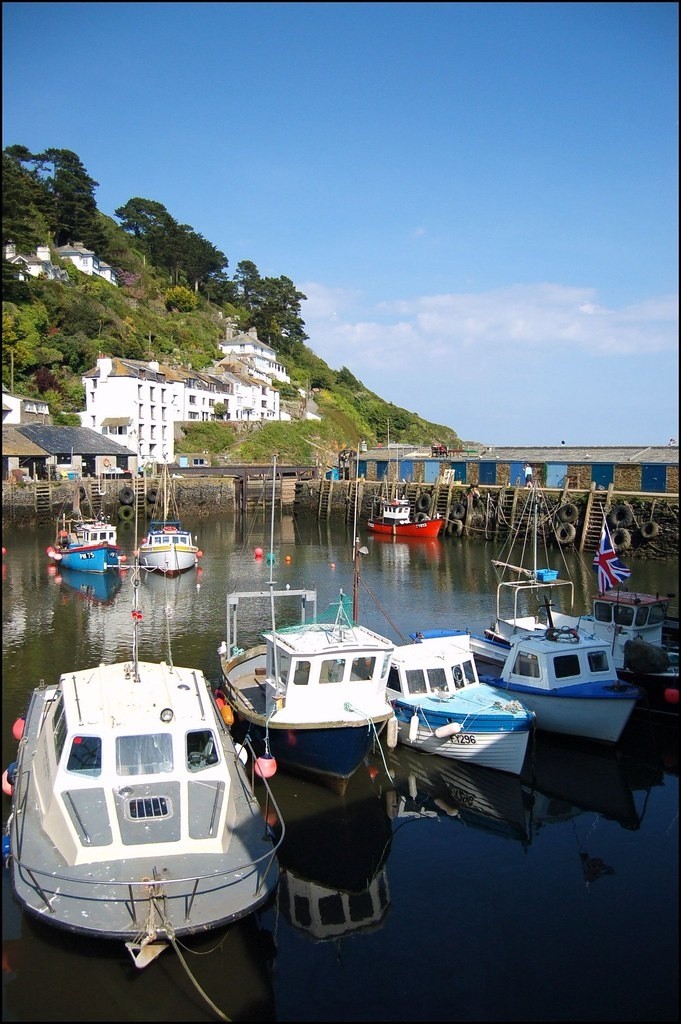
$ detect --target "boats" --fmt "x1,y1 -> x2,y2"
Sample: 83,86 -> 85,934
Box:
46,466 -> 129,573
0,468 -> 287,973
387,627 -> 540,777
468,468 -> 646,750
54,561 -> 124,603
137,450 -> 198,578
219,578 -> 397,796
520,734 -> 646,889
574,586 -> 680,734
365,473 -> 447,538
241,753 -> 397,970
394,742 -> 534,847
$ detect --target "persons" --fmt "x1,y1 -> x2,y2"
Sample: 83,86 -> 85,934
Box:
525,464 -> 532,488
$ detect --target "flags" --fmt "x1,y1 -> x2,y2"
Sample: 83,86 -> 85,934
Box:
592,525 -> 632,595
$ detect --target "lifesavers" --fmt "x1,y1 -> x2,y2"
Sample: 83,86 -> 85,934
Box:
448,518 -> 463,536
452,504 -> 466,519
119,486 -> 134,505
118,505 -> 134,521
640,521 -> 659,539
71,486 -> 86,502
555,522 -> 576,544
609,527 -> 631,553
121,521 -> 134,534
417,493 -> 432,512
147,503 -> 160,519
104,458 -> 110,467
413,512 -> 428,522
560,504 -> 579,522
147,489 -> 158,503
608,504 -> 633,528
